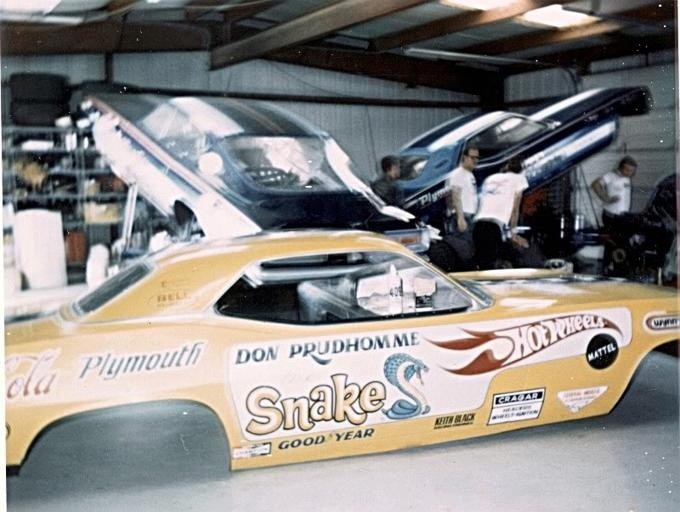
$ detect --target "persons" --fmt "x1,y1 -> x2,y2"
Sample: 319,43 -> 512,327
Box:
592,156 -> 636,273
444,144 -> 480,271
471,160 -> 530,269
369,155 -> 406,208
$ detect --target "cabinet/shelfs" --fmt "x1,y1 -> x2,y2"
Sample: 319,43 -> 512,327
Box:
1,126 -> 130,265
